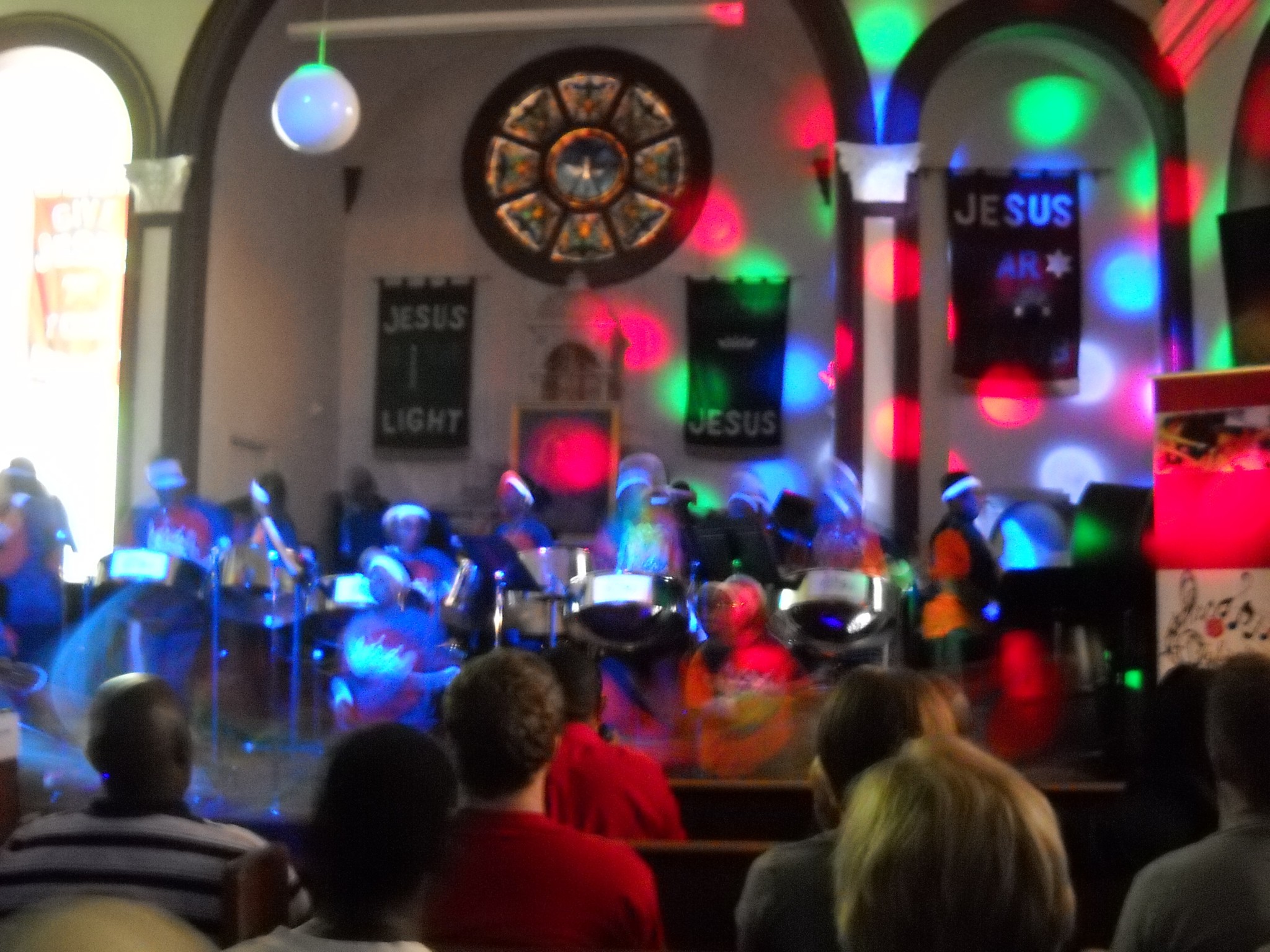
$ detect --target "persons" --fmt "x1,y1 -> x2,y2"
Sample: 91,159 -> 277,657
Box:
0,448 -> 1270,951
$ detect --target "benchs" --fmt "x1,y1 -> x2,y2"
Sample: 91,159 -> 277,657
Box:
637,776 -> 1125,952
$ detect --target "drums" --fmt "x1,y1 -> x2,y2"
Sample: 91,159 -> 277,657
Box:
104,538 -> 901,654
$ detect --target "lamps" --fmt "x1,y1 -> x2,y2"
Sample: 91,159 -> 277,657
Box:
271,0 -> 362,155
